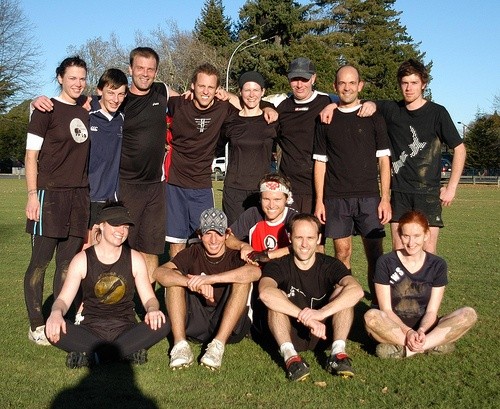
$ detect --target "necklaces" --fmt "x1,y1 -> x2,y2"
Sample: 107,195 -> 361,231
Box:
203,246 -> 227,264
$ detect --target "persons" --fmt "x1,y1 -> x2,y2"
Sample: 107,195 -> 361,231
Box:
24,55 -> 91,346
225,173 -> 300,339
319,58 -> 466,256
76,47 -> 193,317
44,202 -> 172,369
312,65 -> 392,310
152,208 -> 262,371
30,68 -> 129,324
363,210 -> 478,360
164,64 -> 279,260
217,71 -> 278,228
258,213 -> 365,381
228,57 -> 340,254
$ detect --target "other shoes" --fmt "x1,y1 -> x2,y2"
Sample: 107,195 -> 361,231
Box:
126,351 -> 148,364
66,351 -> 89,370
376,343 -> 406,360
28,324 -> 51,346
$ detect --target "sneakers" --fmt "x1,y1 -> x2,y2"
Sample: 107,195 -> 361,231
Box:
201,339 -> 224,371
285,355 -> 310,383
169,341 -> 193,371
327,353 -> 355,376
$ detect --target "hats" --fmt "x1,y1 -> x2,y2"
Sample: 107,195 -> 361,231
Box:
288,58 -> 315,81
98,205 -> 136,227
200,206 -> 227,236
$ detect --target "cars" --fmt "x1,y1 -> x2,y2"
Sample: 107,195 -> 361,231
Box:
441,159 -> 455,178
212,157 -> 226,173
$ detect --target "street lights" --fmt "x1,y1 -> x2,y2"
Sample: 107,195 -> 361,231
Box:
225,34 -> 259,93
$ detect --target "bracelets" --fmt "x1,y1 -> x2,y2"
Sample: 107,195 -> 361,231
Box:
27,189 -> 37,197
406,329 -> 414,335
